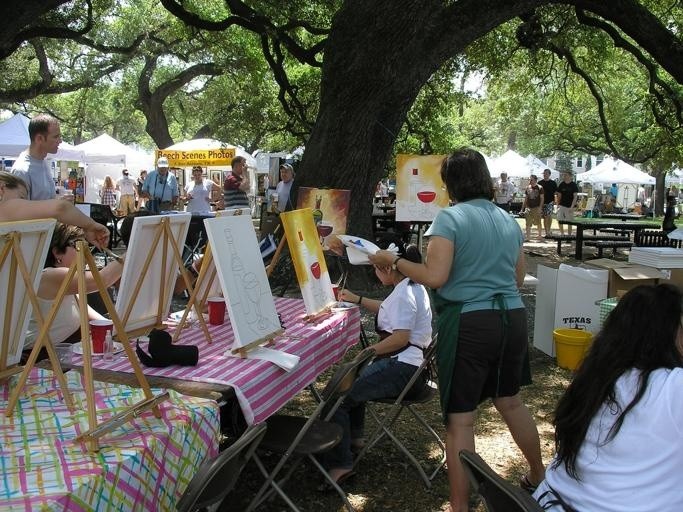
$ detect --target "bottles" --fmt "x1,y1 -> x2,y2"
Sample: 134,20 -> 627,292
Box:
62,189 -> 74,204
103,330 -> 114,362
297,229 -> 316,294
407,168 -> 423,216
310,193 -> 322,233
375,199 -> 384,213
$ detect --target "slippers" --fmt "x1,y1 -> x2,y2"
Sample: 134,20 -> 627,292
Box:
521,476 -> 545,490
318,470 -> 357,491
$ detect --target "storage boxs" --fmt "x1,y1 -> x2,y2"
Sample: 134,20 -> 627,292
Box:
587,258 -> 666,298
658,269 -> 683,296
533,261 -> 609,359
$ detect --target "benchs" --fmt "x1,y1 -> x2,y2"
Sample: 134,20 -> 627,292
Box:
75,202 -> 126,248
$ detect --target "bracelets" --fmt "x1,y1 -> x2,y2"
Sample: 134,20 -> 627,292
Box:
357,295 -> 362,304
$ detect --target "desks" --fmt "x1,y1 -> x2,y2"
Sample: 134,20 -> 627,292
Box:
372,205 -> 415,244
40,294 -> 361,443
0,366 -> 224,512
543,213 -> 661,260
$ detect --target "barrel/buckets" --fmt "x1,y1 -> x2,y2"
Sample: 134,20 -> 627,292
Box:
553,328 -> 594,370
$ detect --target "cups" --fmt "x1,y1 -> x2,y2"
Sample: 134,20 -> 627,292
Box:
144,195 -> 158,212
88,319 -> 113,353
207,297 -> 225,325
332,283 -> 339,296
52,343 -> 72,374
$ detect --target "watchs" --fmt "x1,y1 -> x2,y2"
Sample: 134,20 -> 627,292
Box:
392,257 -> 401,272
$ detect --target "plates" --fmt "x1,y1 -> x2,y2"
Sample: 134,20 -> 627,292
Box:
334,234 -> 381,256
70,341 -> 125,356
330,301 -> 353,309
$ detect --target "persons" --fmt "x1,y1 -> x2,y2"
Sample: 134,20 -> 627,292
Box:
1,113 -> 297,363
637,184 -> 647,207
520,175 -> 545,241
678,188 -> 683,204
368,147 -> 545,512
537,169 -> 559,234
531,282 -> 682,511
316,231 -> 432,494
610,183 -> 618,201
492,172 -> 517,213
555,173 -> 580,235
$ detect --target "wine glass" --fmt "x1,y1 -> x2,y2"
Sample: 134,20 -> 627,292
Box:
316,221 -> 334,251
416,186 -> 436,218
310,260 -> 325,295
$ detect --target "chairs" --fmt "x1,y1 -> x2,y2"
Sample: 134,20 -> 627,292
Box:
458,449 -> 546,512
176,422 -> 266,512
245,346 -> 375,511
351,333 -> 447,488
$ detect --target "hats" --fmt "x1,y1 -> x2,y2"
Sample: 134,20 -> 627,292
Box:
280,163 -> 294,171
159,157 -> 168,167
